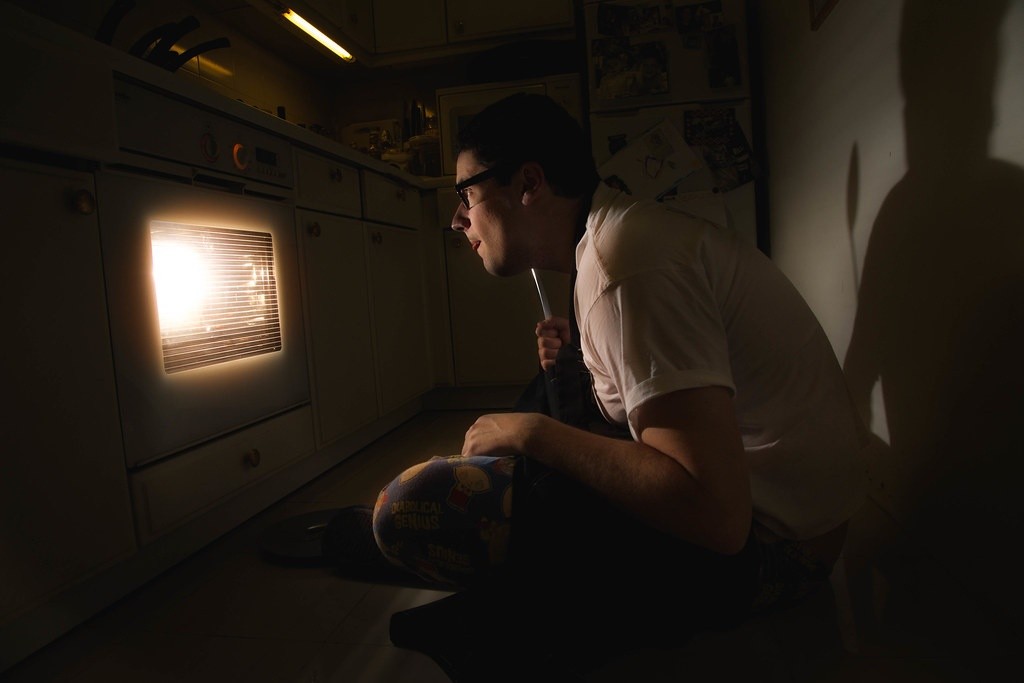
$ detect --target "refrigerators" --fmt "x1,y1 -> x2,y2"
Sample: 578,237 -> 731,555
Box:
583,0 -> 756,247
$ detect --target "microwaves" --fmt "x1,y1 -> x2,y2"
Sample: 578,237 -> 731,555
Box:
435,73 -> 585,176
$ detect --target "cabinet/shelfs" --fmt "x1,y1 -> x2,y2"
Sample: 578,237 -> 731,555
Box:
290,146 -> 434,452
304,0 -> 574,54
0,29 -> 317,673
436,187 -> 572,386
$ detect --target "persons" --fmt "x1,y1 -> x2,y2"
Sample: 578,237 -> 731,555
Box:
373,91 -> 867,640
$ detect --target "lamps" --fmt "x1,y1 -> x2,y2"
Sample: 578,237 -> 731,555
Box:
279,6 -> 358,65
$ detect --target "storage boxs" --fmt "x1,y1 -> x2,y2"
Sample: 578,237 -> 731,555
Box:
336,98 -> 409,153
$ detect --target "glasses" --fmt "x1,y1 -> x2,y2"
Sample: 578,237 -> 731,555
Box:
453,158 -> 522,207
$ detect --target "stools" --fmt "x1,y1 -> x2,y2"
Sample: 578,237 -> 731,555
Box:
389,591 -> 842,683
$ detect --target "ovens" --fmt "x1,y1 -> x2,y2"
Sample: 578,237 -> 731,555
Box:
95,77 -> 312,470
